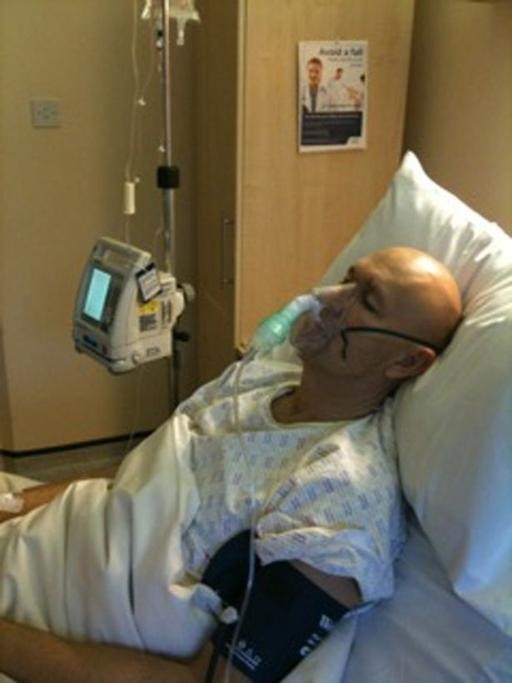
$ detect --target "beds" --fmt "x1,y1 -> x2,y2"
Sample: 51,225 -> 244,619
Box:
0,471 -> 512,683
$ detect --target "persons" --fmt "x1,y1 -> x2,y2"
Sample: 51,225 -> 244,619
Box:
1,246 -> 463,679
355,74 -> 364,95
328,66 -> 354,92
302,58 -> 328,113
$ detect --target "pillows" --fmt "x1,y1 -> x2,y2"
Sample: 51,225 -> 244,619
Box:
270,150 -> 511,636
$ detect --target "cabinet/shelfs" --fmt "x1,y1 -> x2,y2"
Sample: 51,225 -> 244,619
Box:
193,0 -> 415,386
